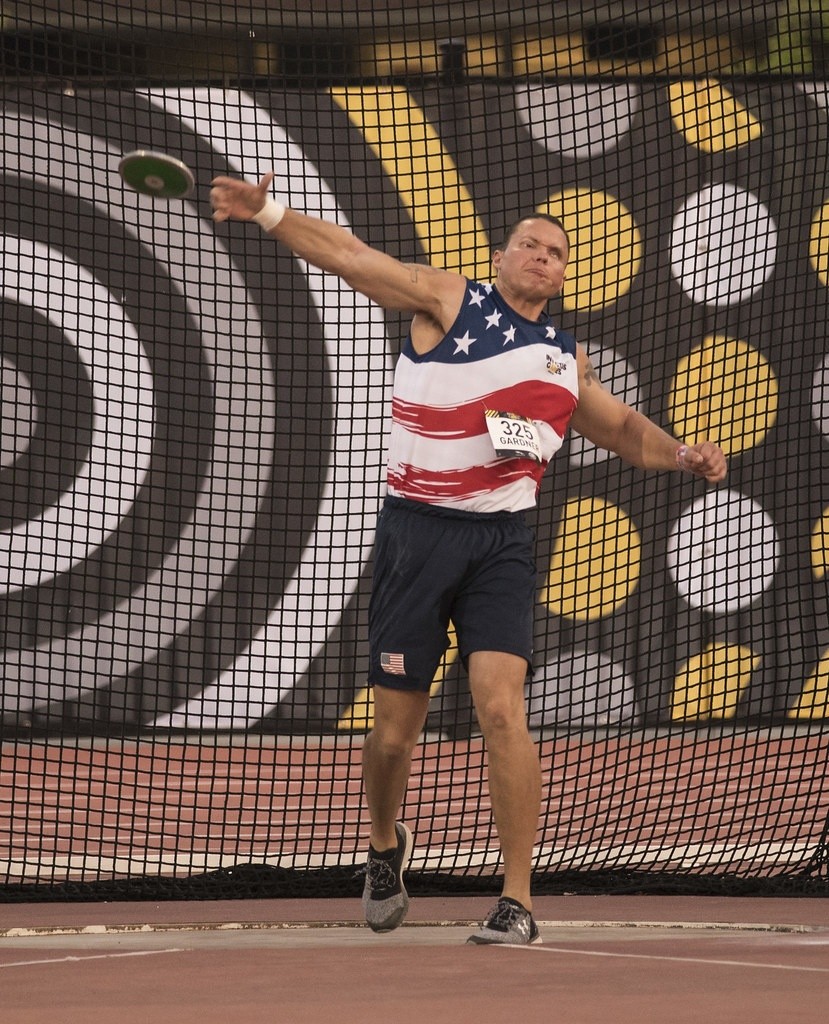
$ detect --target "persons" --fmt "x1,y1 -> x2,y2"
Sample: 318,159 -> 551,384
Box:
209,173 -> 726,944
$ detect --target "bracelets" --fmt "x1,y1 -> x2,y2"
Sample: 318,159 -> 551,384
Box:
675,446 -> 688,471
251,196 -> 285,232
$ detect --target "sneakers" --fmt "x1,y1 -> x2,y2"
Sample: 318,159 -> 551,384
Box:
362,823 -> 416,931
465,898 -> 546,945
